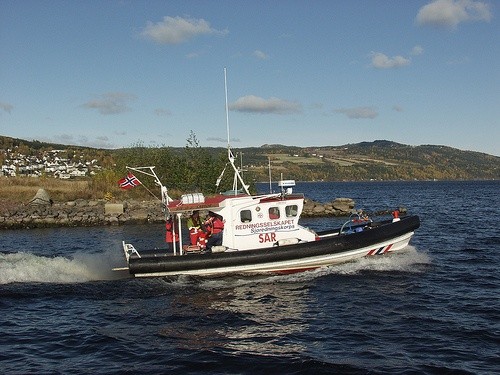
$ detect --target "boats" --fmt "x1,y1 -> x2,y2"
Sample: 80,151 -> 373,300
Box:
117,68 -> 421,279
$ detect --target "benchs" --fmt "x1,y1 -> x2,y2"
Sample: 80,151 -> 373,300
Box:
183,245 -> 201,255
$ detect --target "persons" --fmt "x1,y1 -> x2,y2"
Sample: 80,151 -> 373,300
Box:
166,210 -> 224,252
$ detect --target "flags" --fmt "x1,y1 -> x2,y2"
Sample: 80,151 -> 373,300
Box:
116,171 -> 141,190
